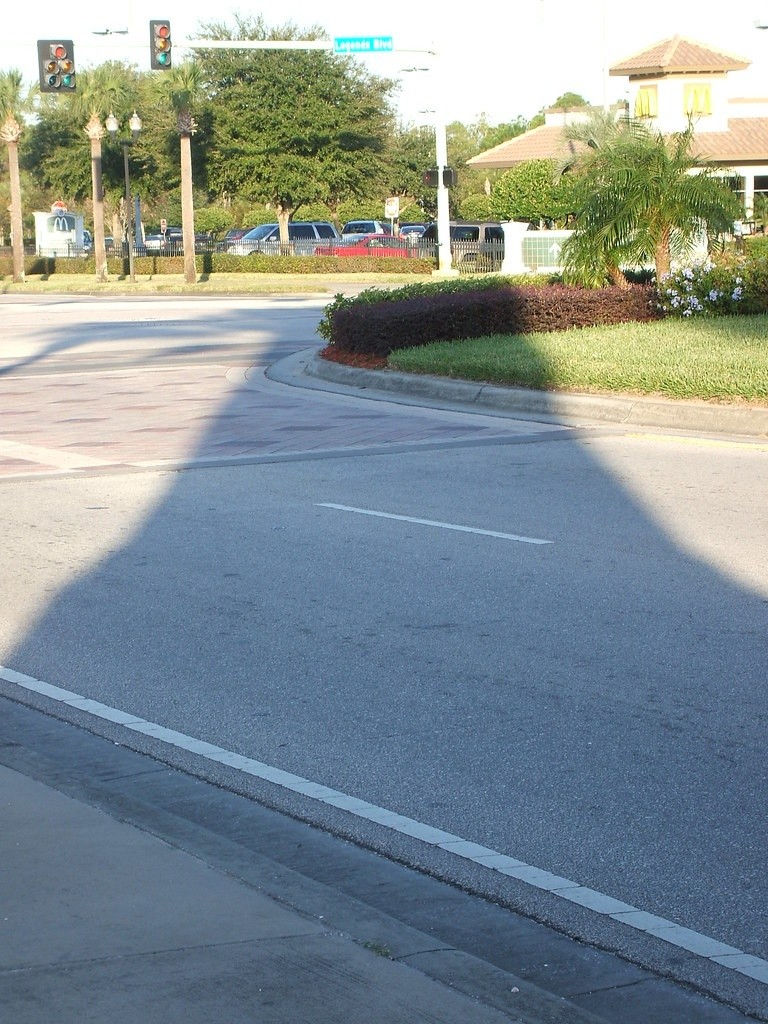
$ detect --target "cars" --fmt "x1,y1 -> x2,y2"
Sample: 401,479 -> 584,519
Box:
105,236 -> 115,255
314,234 -> 418,260
338,220 -> 504,273
122,226 -> 221,257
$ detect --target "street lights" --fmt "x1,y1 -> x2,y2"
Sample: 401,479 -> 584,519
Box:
106,108 -> 139,284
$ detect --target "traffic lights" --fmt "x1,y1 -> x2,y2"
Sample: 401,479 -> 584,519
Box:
442,168 -> 453,187
426,170 -> 438,188
37,39 -> 77,92
149,19 -> 172,71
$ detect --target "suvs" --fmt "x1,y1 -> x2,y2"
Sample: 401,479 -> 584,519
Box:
225,222 -> 344,259
82,230 -> 93,254
